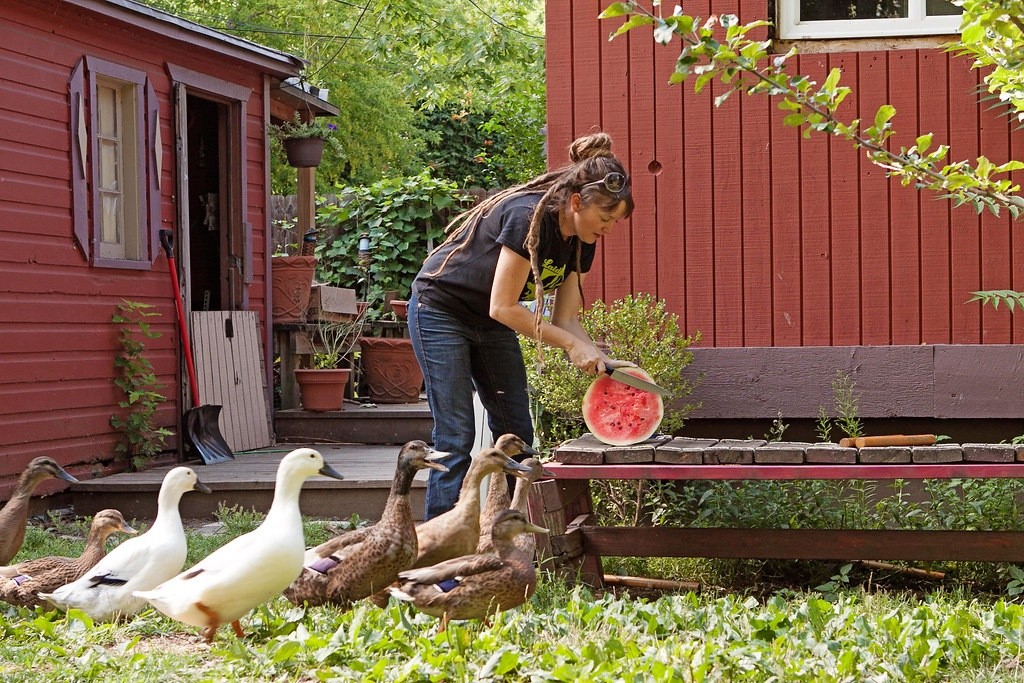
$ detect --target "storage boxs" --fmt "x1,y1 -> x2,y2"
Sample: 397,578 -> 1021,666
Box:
308,286 -> 359,324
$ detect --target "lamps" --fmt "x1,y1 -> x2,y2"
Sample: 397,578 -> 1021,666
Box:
304,226 -> 319,241
358,232 -> 371,251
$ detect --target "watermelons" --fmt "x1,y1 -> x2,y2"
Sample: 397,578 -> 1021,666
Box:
581,368 -> 664,446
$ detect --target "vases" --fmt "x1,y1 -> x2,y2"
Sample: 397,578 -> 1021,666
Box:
272,255 -> 317,322
355,338 -> 424,404
356,301 -> 369,325
390,298 -> 409,319
280,138 -> 329,168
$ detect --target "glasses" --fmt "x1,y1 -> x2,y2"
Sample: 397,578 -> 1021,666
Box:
577,172 -> 632,194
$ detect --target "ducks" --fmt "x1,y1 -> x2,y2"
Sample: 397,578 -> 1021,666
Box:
131,448 -> 345,646
411,430 -> 558,561
0,509 -> 141,610
37,466 -> 211,626
280,440 -> 452,619
0,457 -> 80,565
386,509 -> 550,641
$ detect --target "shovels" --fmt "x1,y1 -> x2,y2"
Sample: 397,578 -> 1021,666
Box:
159,227 -> 236,466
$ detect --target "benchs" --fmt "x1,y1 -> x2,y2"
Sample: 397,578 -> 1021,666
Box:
273,318 -> 411,410
527,343 -> 1024,597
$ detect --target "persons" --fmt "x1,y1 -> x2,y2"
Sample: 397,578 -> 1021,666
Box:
407,133 -> 637,522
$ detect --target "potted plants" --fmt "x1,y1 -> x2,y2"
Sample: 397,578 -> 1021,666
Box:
295,292 -> 380,411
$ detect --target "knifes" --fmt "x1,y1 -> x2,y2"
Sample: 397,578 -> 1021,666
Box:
587,358 -> 674,398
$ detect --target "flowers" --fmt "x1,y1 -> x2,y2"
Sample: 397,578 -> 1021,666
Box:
266,111 -> 338,140
